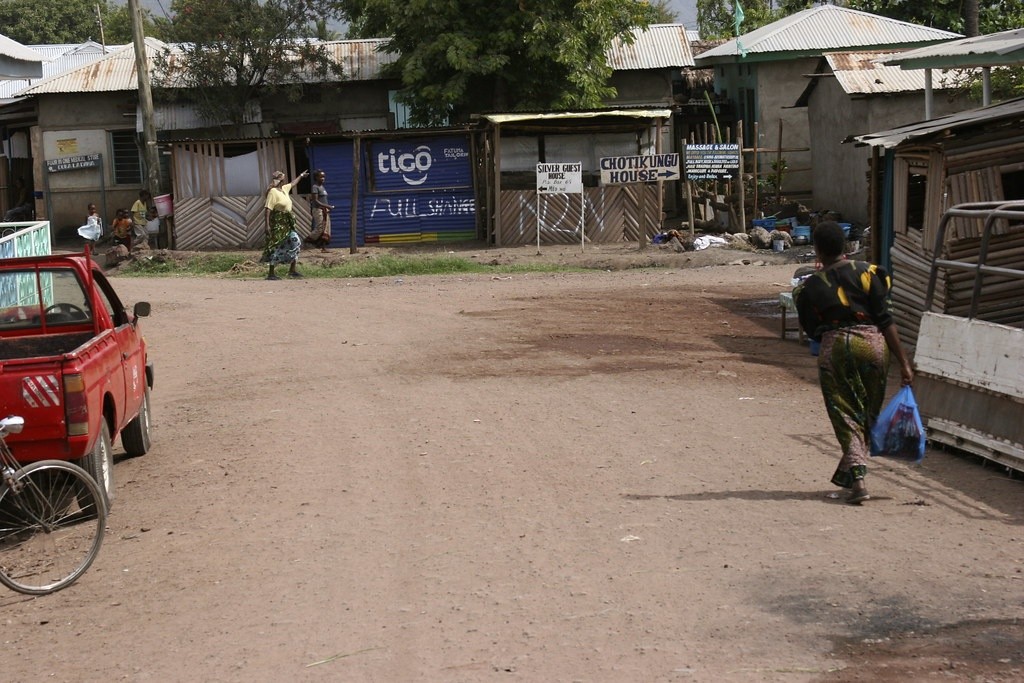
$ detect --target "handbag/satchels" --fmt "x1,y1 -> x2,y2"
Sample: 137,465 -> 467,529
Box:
869,384 -> 925,463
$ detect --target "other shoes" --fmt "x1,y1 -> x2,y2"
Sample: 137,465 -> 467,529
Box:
846,488 -> 870,503
289,272 -> 302,277
92,252 -> 99,255
265,276 -> 281,281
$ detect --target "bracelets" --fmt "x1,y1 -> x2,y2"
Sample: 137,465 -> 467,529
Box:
300,173 -> 304,179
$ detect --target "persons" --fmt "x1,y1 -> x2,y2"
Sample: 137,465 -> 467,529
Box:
302,168 -> 335,253
264,169 -> 309,280
86,189 -> 160,255
3,177 -> 34,222
797,220 -> 915,504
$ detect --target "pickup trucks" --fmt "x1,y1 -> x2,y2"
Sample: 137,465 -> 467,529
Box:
0,243 -> 155,516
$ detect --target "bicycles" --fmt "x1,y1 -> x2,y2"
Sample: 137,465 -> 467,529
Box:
0,415 -> 107,596
